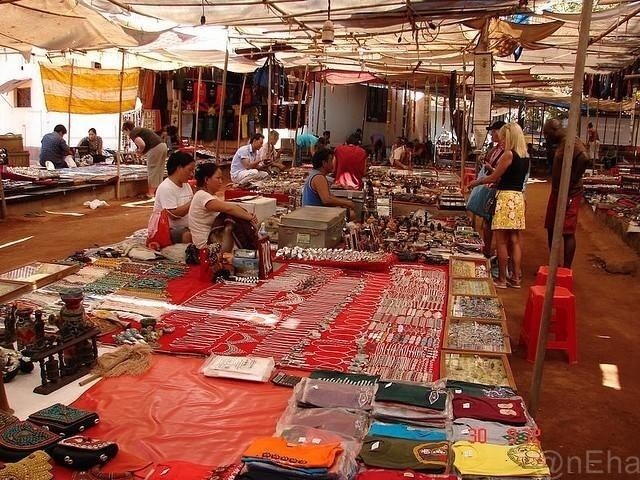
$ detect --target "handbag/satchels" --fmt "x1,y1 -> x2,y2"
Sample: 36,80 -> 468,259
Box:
53,435 -> 120,471
466,163 -> 497,221
0,421 -> 65,463
25,402 -> 100,437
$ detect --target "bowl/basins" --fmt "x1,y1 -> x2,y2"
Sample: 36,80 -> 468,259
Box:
233,258 -> 259,269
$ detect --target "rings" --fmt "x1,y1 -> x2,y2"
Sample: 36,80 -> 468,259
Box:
442,257 -> 509,391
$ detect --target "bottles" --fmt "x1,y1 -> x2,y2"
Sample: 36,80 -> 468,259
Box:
257,223 -> 268,239
33,310 -> 45,339
16,309 -> 37,351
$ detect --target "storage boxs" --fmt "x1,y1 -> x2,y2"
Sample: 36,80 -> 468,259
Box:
277,205 -> 348,250
224,196 -> 277,222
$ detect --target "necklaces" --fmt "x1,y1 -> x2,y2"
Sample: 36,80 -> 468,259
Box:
0,254 -> 391,374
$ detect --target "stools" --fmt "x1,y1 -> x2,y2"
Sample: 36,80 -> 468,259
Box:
519,285 -> 582,366
535,266 -> 574,290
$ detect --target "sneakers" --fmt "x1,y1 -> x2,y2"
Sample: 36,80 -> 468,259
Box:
491,256 -> 522,289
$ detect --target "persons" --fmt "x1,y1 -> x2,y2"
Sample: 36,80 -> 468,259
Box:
463,121 -> 529,289
371,133 -> 387,165
122,118 -> 178,198
146,151 -> 196,244
587,122 -> 601,161
78,128 -> 102,162
294,128 -> 367,223
255,130 -> 286,173
230,133 -> 269,189
390,135 -> 415,171
543,119 -> 589,267
39,125 -> 75,168
188,162 -> 260,254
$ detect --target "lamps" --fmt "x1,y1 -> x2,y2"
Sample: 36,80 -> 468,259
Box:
322,0 -> 334,42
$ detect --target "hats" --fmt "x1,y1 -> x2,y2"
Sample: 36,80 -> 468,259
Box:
486,121 -> 506,130
